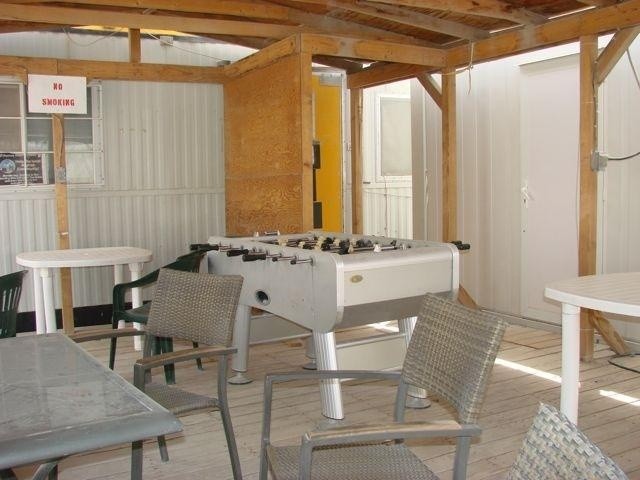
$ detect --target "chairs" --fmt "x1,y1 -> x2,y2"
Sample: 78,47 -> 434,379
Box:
506,402 -> 630,480
109,243 -> 220,385
0,269 -> 28,339
259,292 -> 506,480
69,268 -> 244,479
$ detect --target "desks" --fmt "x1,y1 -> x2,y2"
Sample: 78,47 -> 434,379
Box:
15,246 -> 153,352
544,272 -> 640,426
0,331 -> 183,480
